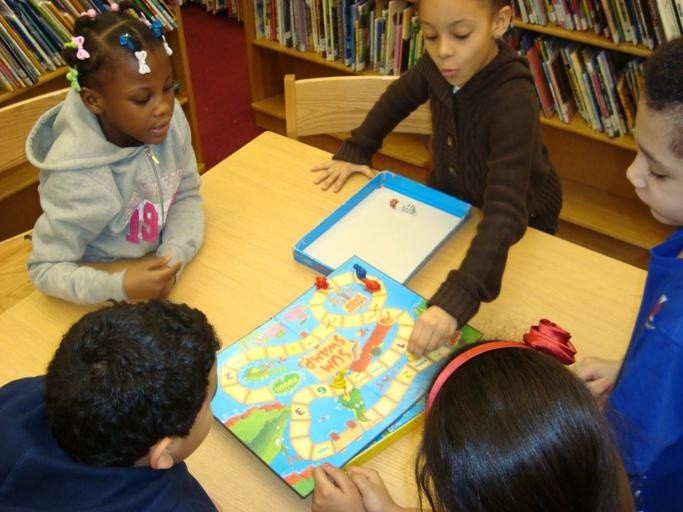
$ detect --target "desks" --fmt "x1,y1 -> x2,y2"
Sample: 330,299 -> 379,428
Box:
1,130 -> 648,512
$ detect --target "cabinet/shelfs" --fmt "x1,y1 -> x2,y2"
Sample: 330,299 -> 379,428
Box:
510,0 -> 683,250
0,0 -> 205,242
243,0 -> 434,185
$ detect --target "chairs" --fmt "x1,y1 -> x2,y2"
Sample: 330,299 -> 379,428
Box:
0,87 -> 71,313
283,73 -> 433,139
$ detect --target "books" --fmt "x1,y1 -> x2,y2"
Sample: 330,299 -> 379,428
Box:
500,1 -> 683,141
0,2 -> 182,96
251,1 -> 426,78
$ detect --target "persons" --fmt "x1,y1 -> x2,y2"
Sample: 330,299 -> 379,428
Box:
309,1 -> 566,360
572,41 -> 681,511
311,337 -> 637,511
23,9 -> 208,311
2,298 -> 227,512
